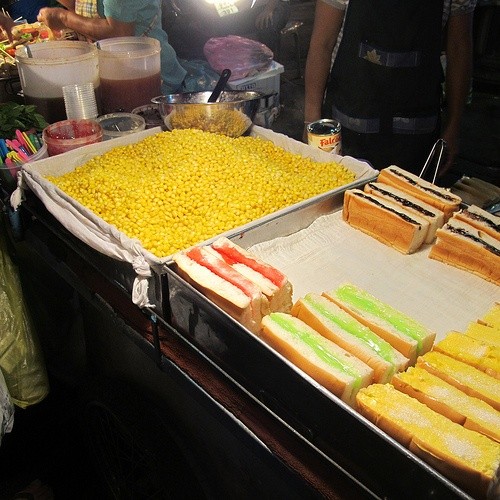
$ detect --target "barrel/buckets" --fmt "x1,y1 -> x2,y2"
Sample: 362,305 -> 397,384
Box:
94,36 -> 162,115
14,41 -> 102,134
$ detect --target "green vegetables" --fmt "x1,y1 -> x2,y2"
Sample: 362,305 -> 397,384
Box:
0,33 -> 31,52
0,102 -> 50,141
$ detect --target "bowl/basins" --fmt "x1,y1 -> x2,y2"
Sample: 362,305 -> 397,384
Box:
131,103 -> 164,129
94,112 -> 146,141
150,89 -> 268,139
42,119 -> 104,157
0,138 -> 49,187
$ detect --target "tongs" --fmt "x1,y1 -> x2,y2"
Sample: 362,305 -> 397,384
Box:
419,139 -> 446,184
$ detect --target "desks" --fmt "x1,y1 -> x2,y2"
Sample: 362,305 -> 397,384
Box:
8,202 -> 373,500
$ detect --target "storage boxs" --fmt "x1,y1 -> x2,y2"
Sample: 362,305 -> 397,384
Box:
205,59 -> 284,113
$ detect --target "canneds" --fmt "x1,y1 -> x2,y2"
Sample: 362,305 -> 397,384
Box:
307,119 -> 343,156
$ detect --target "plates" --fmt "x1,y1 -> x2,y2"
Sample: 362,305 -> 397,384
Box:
10,24 -> 66,40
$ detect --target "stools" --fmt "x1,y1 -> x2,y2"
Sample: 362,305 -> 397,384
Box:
281,18 -> 304,79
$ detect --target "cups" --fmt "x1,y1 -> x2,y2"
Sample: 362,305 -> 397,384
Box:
62,83 -> 99,122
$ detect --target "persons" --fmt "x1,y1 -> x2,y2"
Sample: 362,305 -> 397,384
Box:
303,0 -> 477,187
0,0 -> 291,97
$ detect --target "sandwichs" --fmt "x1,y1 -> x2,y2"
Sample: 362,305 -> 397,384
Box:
262,281 -> 500,500
174,237 -> 293,338
342,165 -> 500,288
